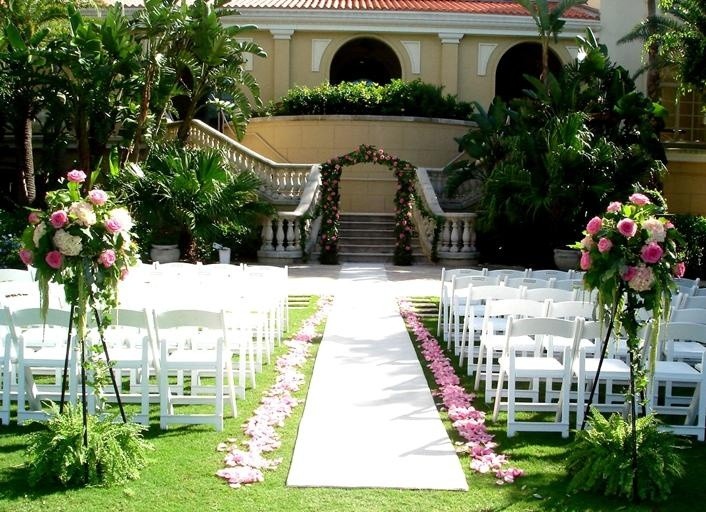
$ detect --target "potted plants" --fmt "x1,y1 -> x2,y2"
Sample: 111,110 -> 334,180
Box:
538,141 -> 582,271
146,141 -> 192,262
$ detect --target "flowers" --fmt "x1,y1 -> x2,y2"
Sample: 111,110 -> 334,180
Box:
19,166 -> 141,339
566,192 -> 687,384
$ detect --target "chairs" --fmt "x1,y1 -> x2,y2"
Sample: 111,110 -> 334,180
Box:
0,261 -> 288,431
436,267 -> 706,440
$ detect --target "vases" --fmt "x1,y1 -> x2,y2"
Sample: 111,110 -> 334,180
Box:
219,247 -> 231,264
